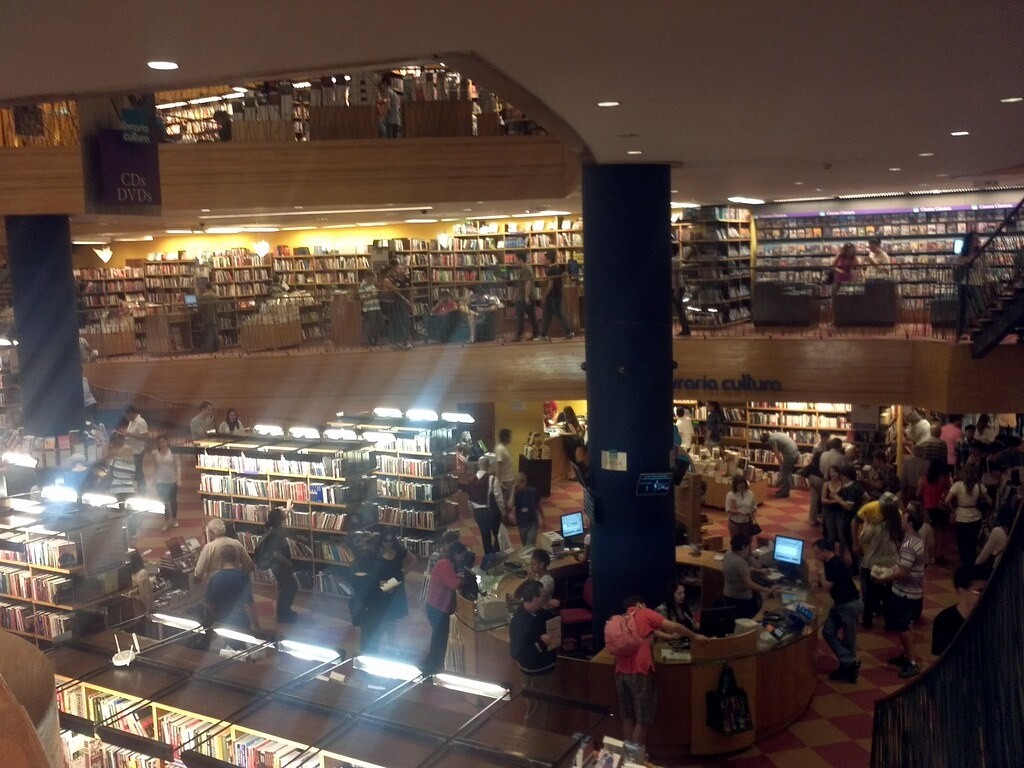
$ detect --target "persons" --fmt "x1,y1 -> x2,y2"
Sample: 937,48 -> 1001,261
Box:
417,530 -> 474,668
358,269 -> 385,348
510,475 -> 546,546
812,538 -> 864,682
204,544 -> 261,635
468,456 -> 509,552
722,531 -> 777,605
117,403 -> 149,496
421,289 -> 459,336
266,273 -> 283,298
604,583 -> 714,750
660,244 -> 689,338
458,286 -> 496,343
397,260 -> 413,351
492,430 -> 512,479
760,432 -> 802,497
217,407 -> 246,435
827,243 -> 859,328
384,260 -> 412,348
531,251 -> 574,343
191,400 -> 216,439
809,404 -> 1023,674
863,237 -> 893,292
199,282 -> 223,352
510,253 -> 541,341
192,517 -> 255,584
953,231 -> 984,338
262,498 -> 302,623
508,548 -> 567,728
561,405 -> 585,481
151,435 -> 181,533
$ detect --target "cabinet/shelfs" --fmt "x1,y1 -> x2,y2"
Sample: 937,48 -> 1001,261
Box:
0,202 -> 1024,368
674,470 -> 729,553
327,417 -> 462,570
161,91 -> 309,142
40,628 -> 581,768
0,499 -> 149,647
191,434 -> 379,596
673,400 -> 904,484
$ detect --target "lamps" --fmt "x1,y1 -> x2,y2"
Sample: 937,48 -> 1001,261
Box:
169,438 -> 334,464
148,609 -> 616,764
15,485 -> 167,520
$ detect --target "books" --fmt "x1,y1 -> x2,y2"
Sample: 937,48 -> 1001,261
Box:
196,424 -> 461,603
80,199 -> 1020,375
54,679 -> 320,768
0,523 -> 88,641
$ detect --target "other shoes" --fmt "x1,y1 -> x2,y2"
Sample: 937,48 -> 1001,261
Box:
859,616 -> 872,628
830,662 -> 860,682
889,655 -> 909,666
279,610 -> 297,623
161,522 -> 170,531
898,660 -> 920,678
417,661 -> 429,675
172,520 -> 179,527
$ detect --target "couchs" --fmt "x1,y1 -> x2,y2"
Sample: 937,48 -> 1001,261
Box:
423,308 -> 493,343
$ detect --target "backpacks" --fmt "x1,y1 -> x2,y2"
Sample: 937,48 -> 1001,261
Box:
253,528 -> 274,570
604,609 -> 644,659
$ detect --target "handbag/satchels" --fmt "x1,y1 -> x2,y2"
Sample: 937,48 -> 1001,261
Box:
418,552 -> 439,612
752,517 -> 762,534
497,523 -> 514,553
706,666 -> 754,737
490,495 -> 501,522
796,462 -> 812,477
462,546 -> 476,568
976,495 -> 993,510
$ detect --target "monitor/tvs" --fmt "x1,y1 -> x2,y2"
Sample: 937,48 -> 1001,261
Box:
463,567 -> 478,600
699,606 -> 736,639
773,535 -> 804,566
560,512 -> 584,539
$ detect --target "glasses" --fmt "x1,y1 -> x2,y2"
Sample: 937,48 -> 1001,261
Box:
383,539 -> 393,543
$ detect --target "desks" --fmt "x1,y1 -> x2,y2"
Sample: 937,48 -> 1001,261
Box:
238,320 -> 303,354
701,476 -> 768,509
80,330 -> 139,362
